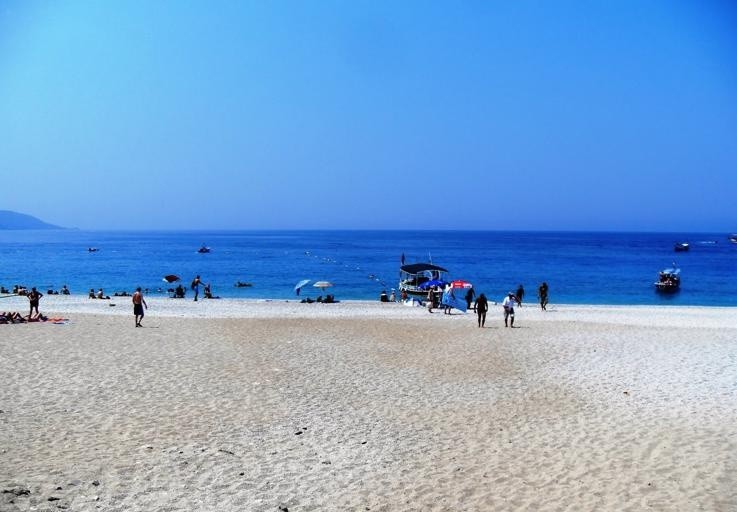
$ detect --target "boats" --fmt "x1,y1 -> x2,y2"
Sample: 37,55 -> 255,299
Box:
87,248 -> 98,251
728,235 -> 737,244
673,242 -> 690,252
198,246 -> 211,254
164,274 -> 181,284
653,266 -> 682,292
234,282 -> 252,288
396,263 -> 452,301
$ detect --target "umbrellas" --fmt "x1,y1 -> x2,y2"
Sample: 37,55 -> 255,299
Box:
451,280 -> 472,288
419,280 -> 450,290
294,280 -> 311,291
313,280 -> 334,292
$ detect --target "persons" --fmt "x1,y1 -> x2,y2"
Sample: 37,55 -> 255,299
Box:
474,293 -> 487,327
427,285 -> 435,312
503,292 -> 517,328
539,282 -> 548,310
0,275 -> 212,327
445,288 -> 455,315
381,289 -> 407,302
466,287 -> 475,309
516,284 -> 524,308
301,294 -> 335,303
